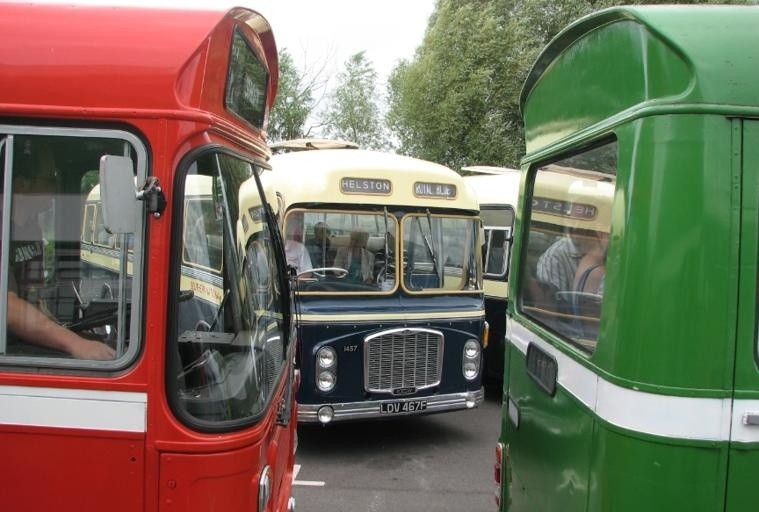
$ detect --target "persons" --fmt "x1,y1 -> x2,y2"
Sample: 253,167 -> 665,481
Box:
373,232 -> 414,281
333,228 -> 376,282
523,178 -> 613,336
7,137 -> 116,360
284,237 -> 313,288
305,222 -> 337,275
